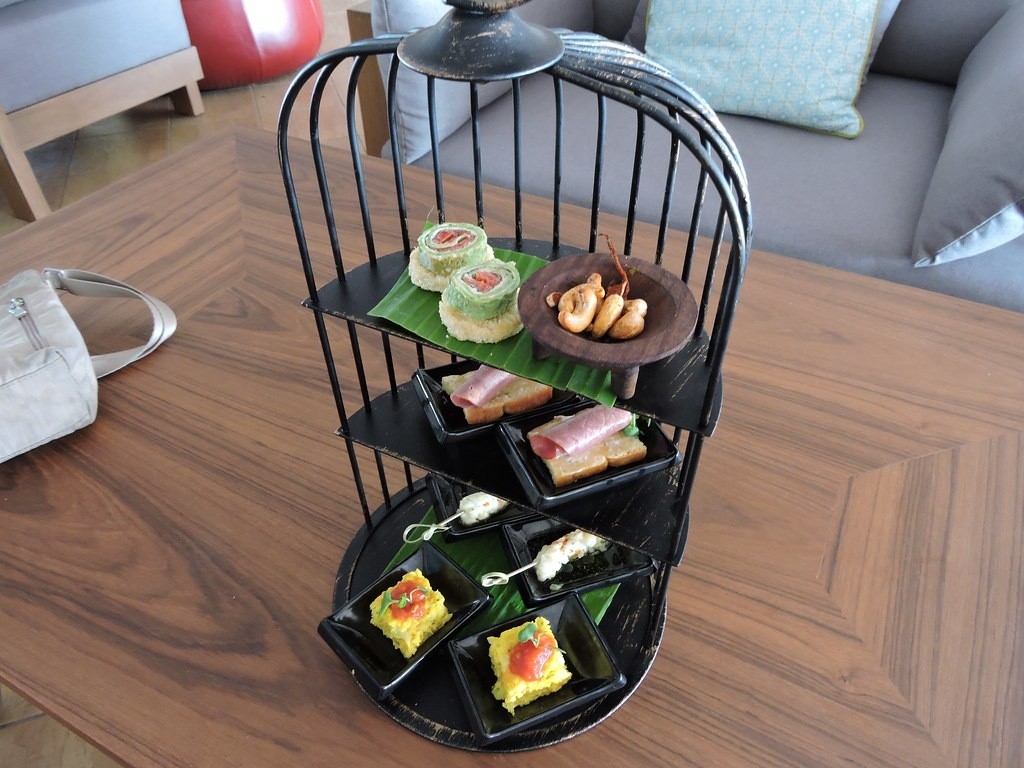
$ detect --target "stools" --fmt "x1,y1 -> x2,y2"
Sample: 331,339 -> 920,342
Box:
180,0 -> 323,92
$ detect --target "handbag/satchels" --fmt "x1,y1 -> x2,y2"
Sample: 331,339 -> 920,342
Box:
0,268 -> 177,462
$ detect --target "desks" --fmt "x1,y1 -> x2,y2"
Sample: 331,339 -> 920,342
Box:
0,123 -> 1024,768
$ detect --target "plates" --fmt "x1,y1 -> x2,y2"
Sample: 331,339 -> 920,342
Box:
316,537 -> 495,702
495,401 -> 682,509
425,472 -> 547,543
443,590 -> 627,746
412,360 -> 598,446
500,514 -> 658,610
516,253 -> 700,402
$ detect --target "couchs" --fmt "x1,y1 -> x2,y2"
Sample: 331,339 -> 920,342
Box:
347,0 -> 1024,314
0,0 -> 206,221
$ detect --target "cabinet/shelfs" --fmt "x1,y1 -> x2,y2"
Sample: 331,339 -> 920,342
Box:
278,0 -> 753,754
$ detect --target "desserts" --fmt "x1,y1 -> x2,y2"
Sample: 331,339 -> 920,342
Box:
486,616 -> 572,717
408,222 -> 524,344
369,568 -> 453,658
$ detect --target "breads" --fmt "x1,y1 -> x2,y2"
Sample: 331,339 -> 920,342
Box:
440,368 -> 553,424
528,414 -> 647,488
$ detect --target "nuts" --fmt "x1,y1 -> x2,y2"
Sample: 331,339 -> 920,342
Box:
546,273 -> 648,340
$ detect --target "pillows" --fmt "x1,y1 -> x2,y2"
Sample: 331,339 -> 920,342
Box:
621,0 -> 902,138
